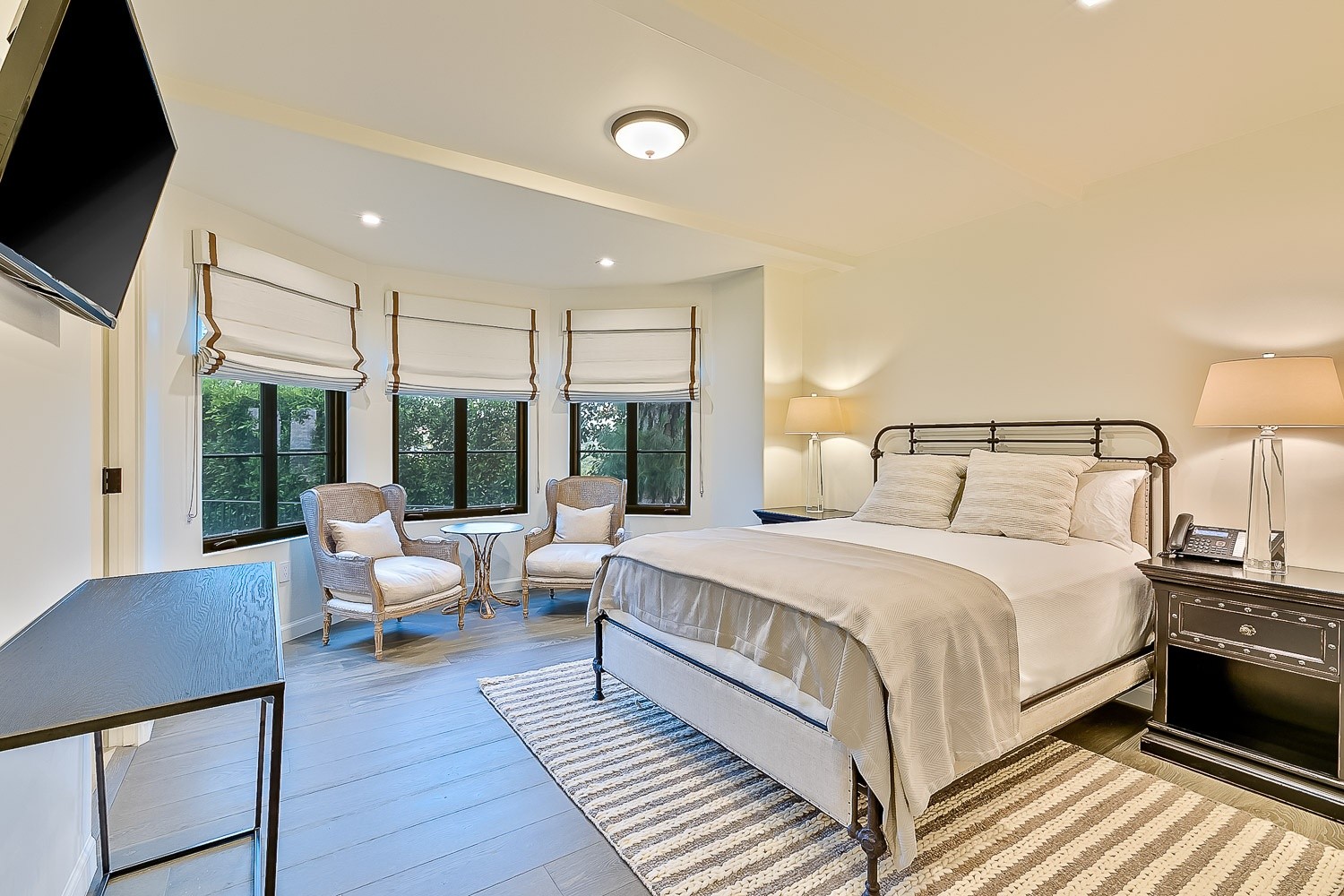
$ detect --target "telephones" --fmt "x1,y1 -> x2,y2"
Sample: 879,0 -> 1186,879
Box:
1166,513 -> 1287,563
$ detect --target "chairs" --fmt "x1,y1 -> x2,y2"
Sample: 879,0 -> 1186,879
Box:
522,475 -> 628,618
300,482 -> 468,661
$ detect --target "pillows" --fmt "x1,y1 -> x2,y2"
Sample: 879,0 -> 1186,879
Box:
326,509 -> 405,560
551,502 -> 614,544
947,449 -> 1099,544
850,452 -> 969,528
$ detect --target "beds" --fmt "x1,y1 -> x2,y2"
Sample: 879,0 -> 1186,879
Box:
585,419 -> 1177,896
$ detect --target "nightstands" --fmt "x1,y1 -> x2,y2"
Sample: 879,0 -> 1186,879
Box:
754,507 -> 856,524
1134,556 -> 1344,824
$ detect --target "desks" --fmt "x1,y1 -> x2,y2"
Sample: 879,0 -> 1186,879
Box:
0,562 -> 286,896
440,522 -> 524,618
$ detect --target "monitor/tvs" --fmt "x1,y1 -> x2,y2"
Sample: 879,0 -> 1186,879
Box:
1,0 -> 177,328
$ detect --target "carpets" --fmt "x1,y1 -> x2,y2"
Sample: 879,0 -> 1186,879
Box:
474,658 -> 1344,896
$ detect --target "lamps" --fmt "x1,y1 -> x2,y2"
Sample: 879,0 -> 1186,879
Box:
1193,354 -> 1344,576
782,393 -> 846,513
611,110 -> 689,159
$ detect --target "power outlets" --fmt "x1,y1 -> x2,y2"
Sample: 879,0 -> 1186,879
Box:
278,561 -> 290,583
